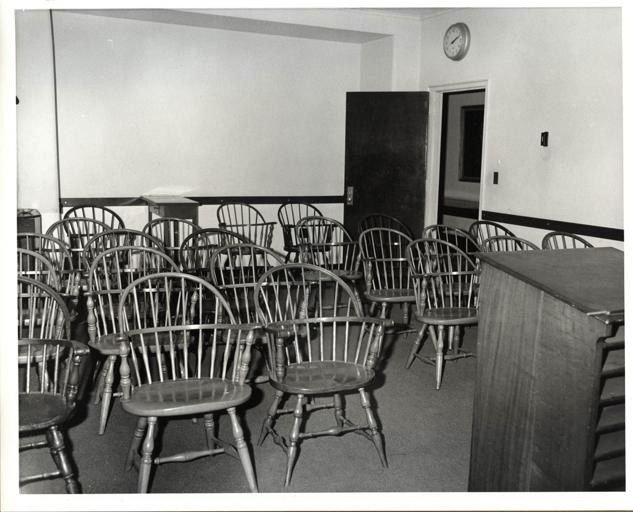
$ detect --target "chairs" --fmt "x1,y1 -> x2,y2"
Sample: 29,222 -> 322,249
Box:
443,23 -> 471,61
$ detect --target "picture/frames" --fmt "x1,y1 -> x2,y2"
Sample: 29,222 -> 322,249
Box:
458,105 -> 484,182
